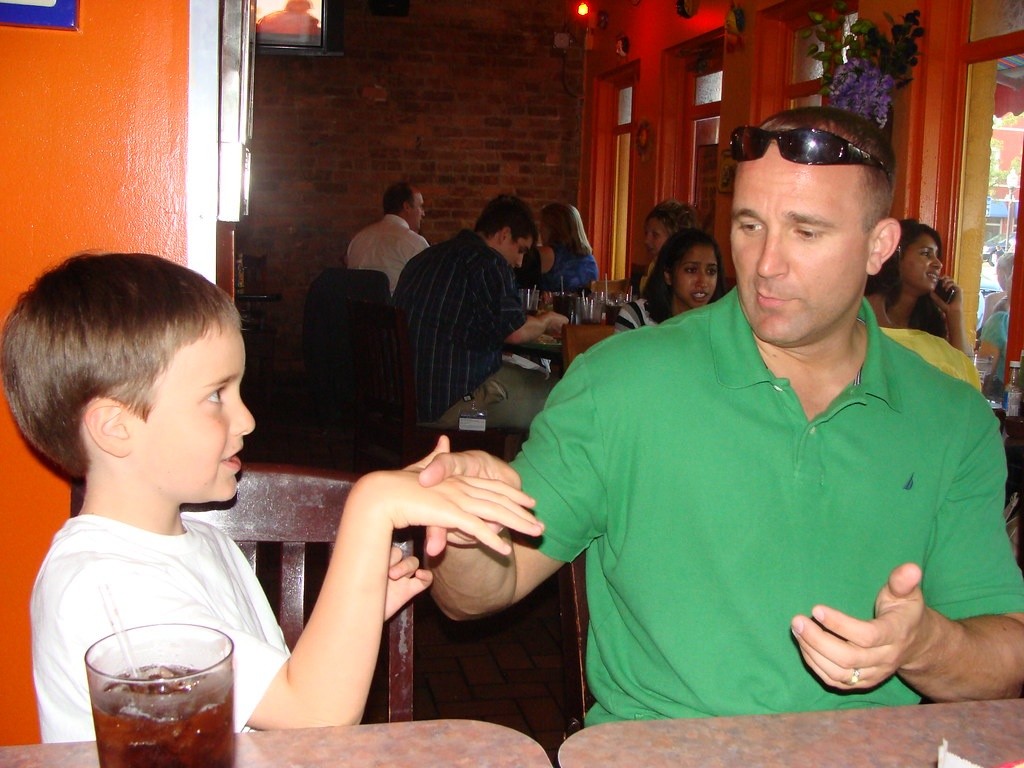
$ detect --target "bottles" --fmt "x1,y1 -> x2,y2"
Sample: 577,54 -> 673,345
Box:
1002,360 -> 1024,428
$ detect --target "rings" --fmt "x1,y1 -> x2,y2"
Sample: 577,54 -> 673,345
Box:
847,668 -> 860,686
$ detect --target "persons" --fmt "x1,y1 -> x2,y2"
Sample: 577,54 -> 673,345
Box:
612,228 -> 727,335
538,201 -> 598,296
346,181 -> 431,297
864,218 -> 974,366
976,244 -> 1015,406
425,107 -> 1024,728
388,193 -> 570,430
0,252 -> 545,744
629,198 -> 701,300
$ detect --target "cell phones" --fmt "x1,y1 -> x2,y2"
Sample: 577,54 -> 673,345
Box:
934,279 -> 955,304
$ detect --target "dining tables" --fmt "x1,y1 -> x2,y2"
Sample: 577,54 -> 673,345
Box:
1,719 -> 550,767
557,700 -> 1022,768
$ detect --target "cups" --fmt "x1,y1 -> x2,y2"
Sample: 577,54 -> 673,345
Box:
515,281 -> 633,328
84,623 -> 238,768
962,351 -> 993,398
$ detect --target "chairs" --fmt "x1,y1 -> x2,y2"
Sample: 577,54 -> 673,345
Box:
302,267 -> 508,468
68,465 -> 415,724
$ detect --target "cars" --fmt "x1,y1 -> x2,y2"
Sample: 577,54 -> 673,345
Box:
982,232 -> 1016,267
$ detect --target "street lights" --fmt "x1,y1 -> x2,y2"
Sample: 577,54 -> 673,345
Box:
1006,168 -> 1017,250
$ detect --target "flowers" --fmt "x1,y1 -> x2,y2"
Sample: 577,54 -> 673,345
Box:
800,0 -> 925,128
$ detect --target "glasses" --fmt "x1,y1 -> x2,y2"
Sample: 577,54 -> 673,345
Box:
728,123 -> 893,188
